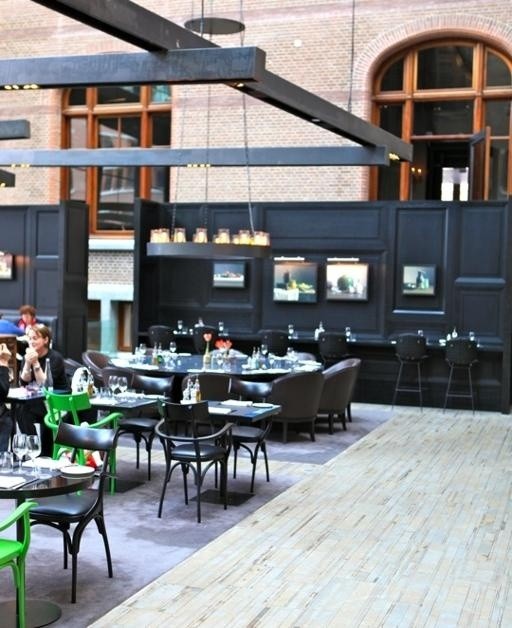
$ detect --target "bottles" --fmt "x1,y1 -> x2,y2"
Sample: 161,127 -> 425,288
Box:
187,378 -> 193,401
194,378 -> 201,402
257,347 -> 261,359
452,328 -> 457,338
320,321 -> 323,329
157,346 -> 162,367
252,347 -> 256,359
152,345 -> 158,366
86,371 -> 95,398
416,270 -> 424,288
43,358 -> 54,394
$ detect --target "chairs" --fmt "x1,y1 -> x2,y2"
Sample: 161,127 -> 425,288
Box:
110,413 -> 174,480
182,374 -> 245,433
318,332 -> 350,366
444,338 -> 479,415
259,328 -> 288,356
17,424 -> 118,605
0,500 -> 38,627
41,389 -> 123,497
220,417 -> 274,492
193,324 -> 216,351
314,360 -> 361,433
128,372 -> 176,418
261,369 -> 325,443
155,417 -> 233,524
392,332 -> 431,409
0,330 -> 18,388
148,324 -> 177,350
102,367 -> 135,392
84,348 -> 115,388
106,407 -> 171,482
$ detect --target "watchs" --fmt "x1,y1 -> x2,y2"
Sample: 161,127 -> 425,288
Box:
33,366 -> 41,372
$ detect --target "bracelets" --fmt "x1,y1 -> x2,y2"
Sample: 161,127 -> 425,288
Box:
23,368 -> 32,375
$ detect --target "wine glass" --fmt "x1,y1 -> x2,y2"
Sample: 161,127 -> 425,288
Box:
267,357 -> 275,371
28,435 -> 41,473
139,343 -> 147,354
118,376 -> 129,401
169,342 -> 176,353
107,375 -> 118,399
286,347 -> 294,357
216,353 -> 223,371
12,433 -> 27,476
261,344 -> 269,355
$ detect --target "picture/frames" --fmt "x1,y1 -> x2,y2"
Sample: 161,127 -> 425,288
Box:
211,258 -> 248,290
0,251 -> 12,280
324,261 -> 370,302
271,259 -> 320,305
401,263 -> 437,297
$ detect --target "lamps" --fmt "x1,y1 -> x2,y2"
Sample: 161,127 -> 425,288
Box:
146,2 -> 271,260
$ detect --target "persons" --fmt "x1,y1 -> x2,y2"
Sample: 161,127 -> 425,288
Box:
0,343 -> 17,454
16,324 -> 68,461
19,305 -> 39,329
0,313 -> 25,337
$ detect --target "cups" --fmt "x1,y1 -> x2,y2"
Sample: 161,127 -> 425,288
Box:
0,451 -> 14,475
100,387 -> 109,399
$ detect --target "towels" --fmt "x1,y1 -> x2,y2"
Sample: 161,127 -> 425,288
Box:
208,407 -> 232,415
90,397 -> 116,405
220,398 -> 253,408
187,369 -> 226,373
0,475 -> 24,489
109,359 -> 131,367
242,368 -> 291,374
21,456 -> 62,470
299,360 -> 322,365
131,363 -> 159,370
116,391 -> 143,399
273,355 -> 296,361
297,364 -> 319,372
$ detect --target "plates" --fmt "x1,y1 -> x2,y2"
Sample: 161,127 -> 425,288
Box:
60,466 -> 95,475
